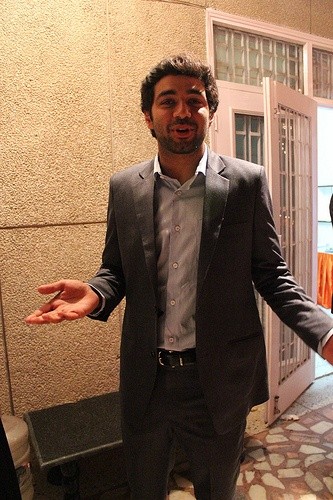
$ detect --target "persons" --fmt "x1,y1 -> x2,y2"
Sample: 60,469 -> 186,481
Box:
23,53 -> 333,500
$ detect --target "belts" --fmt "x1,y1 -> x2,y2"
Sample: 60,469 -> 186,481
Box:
157,347 -> 197,368
